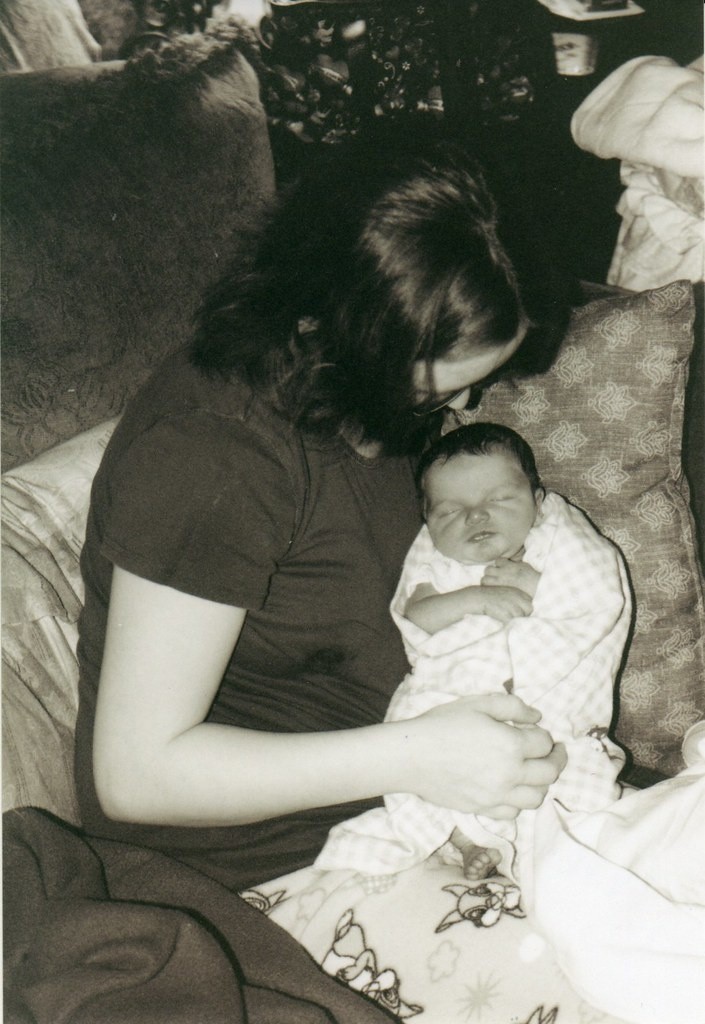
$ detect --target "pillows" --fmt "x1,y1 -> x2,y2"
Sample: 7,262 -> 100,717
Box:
444,281 -> 705,779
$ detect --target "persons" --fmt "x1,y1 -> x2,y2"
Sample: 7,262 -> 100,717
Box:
312,419 -> 632,881
70,132 -> 623,1023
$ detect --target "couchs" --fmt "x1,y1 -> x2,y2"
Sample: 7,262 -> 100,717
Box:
3,35 -> 705,1024
